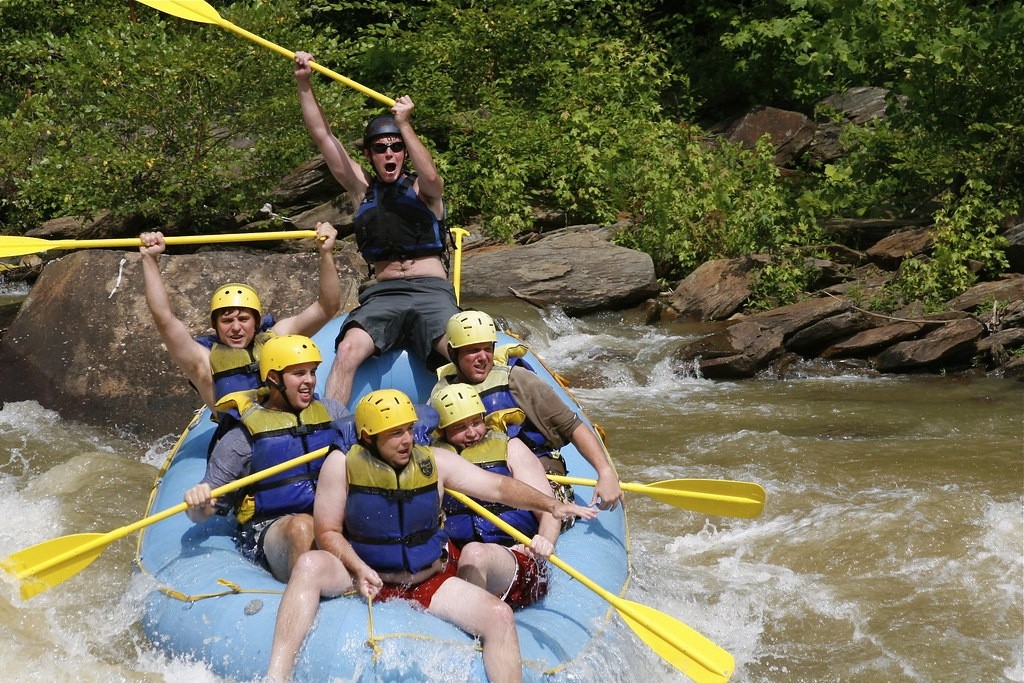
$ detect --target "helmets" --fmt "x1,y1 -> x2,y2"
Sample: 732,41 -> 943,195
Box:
431,382 -> 487,429
362,116 -> 402,148
353,388 -> 418,440
210,283 -> 262,330
445,310 -> 498,348
260,335 -> 322,383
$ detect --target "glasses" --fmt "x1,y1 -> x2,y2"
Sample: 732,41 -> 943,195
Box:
370,141 -> 405,154
443,415 -> 483,432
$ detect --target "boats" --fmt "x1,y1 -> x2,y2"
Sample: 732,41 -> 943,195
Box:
126,302 -> 634,683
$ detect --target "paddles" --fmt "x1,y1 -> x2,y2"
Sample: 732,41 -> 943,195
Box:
128,0 -> 400,110
444,225 -> 473,311
544,474 -> 771,522
0,442 -> 333,602
442,483 -> 740,683
0,224 -> 332,265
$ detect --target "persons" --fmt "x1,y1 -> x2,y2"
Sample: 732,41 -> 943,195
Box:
265,389 -> 601,683
427,383 -> 562,611
139,221 -> 342,424
294,51 -> 463,407
187,334 -> 353,583
426,310 -> 625,530
